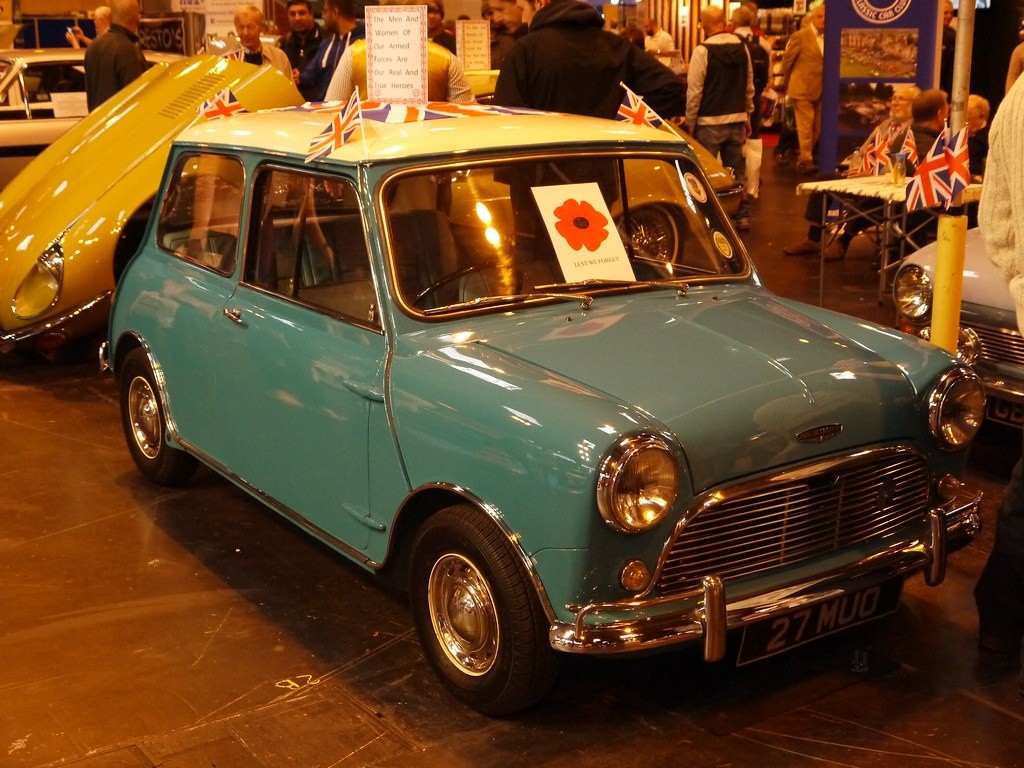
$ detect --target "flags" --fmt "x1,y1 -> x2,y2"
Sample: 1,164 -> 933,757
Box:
900,125 -> 971,213
857,127 -> 890,175
305,91 -> 360,164
196,86 -> 250,120
224,50 -> 248,62
615,93 -> 662,129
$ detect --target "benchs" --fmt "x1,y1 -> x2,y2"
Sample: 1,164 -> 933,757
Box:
161,208 -> 465,312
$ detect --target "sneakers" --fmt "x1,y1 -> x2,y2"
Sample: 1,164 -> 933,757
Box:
783,237 -> 821,254
819,237 -> 847,260
735,215 -> 751,230
798,160 -> 819,174
775,152 -> 789,163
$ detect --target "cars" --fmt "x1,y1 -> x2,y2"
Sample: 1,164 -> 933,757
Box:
888,226 -> 1023,433
0,45 -> 744,359
99,89 -> 989,717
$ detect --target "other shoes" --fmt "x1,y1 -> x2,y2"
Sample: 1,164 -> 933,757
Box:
978,629 -> 1024,653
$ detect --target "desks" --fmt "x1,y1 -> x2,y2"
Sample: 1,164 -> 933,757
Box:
796,174 -> 982,331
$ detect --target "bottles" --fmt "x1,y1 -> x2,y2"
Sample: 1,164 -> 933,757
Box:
894,154 -> 906,187
850,147 -> 863,167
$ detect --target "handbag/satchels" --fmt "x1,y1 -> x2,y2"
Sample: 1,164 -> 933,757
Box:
713,138 -> 763,200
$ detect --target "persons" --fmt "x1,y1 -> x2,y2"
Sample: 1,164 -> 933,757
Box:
301,0 -> 481,216
971,40 -> 1024,688
783,85 -> 922,260
469,0 -> 687,252
940,0 -> 982,98
685,5 -> 755,231
888,85 -> 983,253
84,0 -> 151,114
728,2 -> 767,38
274,0 -> 324,88
781,4 -> 824,172
871,94 -> 990,270
65,6 -> 111,48
223,5 -> 297,90
728,7 -> 774,192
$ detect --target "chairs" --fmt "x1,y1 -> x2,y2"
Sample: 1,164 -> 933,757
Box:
288,278 -> 380,323
459,253 -> 556,302
